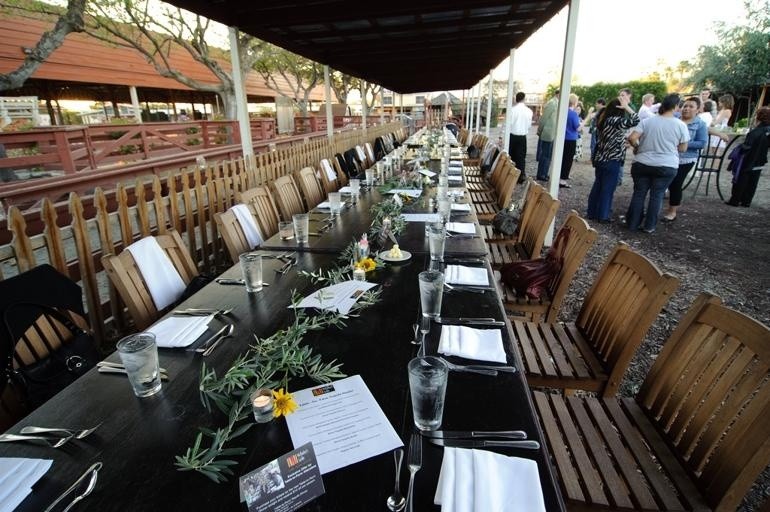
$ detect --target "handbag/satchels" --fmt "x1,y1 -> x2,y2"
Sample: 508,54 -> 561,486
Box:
467,145 -> 479,159
493,208 -> 520,236
13,329 -> 108,406
500,258 -> 555,300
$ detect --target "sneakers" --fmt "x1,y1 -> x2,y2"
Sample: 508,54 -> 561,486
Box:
643,227 -> 655,233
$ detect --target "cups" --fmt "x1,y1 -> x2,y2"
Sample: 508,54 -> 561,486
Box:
406,356 -> 449,432
436,126 -> 452,224
418,269 -> 445,319
238,251 -> 263,296
428,226 -> 446,263
277,124 -> 427,245
115,330 -> 163,400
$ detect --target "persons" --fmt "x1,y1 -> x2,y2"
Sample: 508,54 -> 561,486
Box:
179,109 -> 192,121
724,107 -> 770,208
584,95 -> 640,223
241,463 -> 285,505
618,92 -> 692,235
642,96 -> 710,223
506,92 -> 534,184
535,83 -> 735,200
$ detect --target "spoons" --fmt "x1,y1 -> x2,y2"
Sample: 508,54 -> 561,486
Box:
193,323 -> 235,359
43,461 -> 105,511
386,446 -> 407,511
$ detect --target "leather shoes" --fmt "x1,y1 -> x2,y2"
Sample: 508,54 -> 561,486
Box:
664,216 -> 677,221
598,218 -> 616,224
724,199 -> 751,207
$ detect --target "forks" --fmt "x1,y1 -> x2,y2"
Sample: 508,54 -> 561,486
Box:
438,353 -> 518,376
420,315 -> 432,361
404,428 -> 424,509
173,305 -> 237,322
1,420 -> 106,449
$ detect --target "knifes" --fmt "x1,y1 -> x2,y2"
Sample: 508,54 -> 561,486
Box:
418,428 -> 541,450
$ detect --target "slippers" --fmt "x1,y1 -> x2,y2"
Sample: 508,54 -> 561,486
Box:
559,183 -> 572,188
